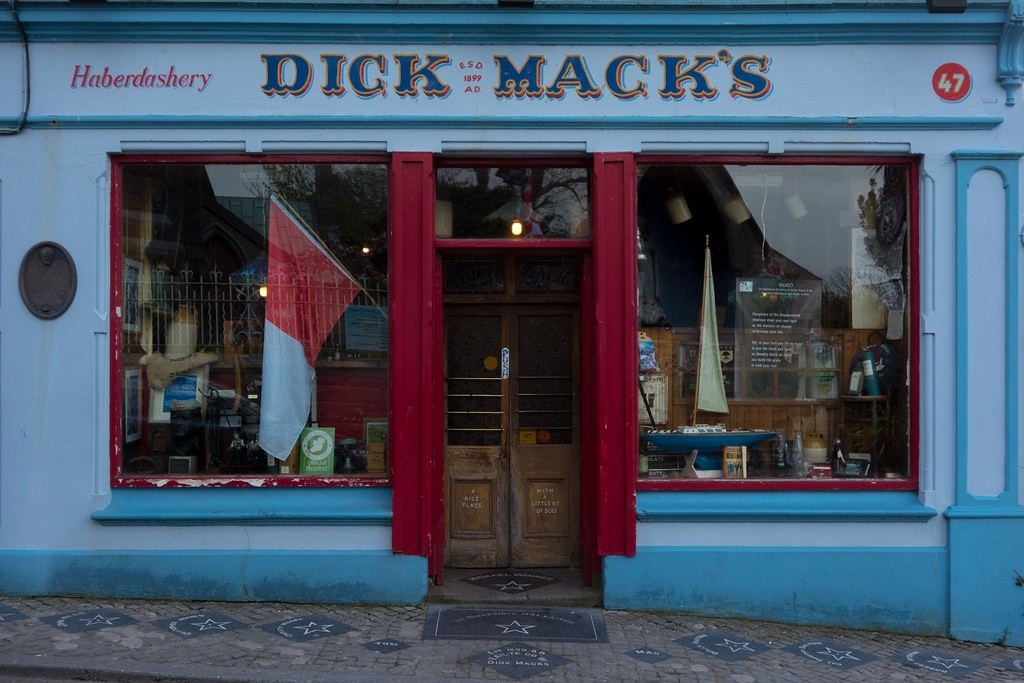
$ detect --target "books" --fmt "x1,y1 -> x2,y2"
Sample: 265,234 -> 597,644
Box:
721,446 -> 749,481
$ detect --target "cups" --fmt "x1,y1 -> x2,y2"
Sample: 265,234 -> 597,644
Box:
804,462 -> 813,478
639,455 -> 648,473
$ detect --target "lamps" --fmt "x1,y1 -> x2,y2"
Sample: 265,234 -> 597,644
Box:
570,169 -> 589,238
663,176 -> 693,226
770,176 -> 808,220
435,168 -> 453,238
699,167 -> 752,225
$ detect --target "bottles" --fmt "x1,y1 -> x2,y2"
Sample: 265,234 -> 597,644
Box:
776,435 -> 787,477
805,434 -> 821,448
848,361 -> 864,395
790,432 -> 803,478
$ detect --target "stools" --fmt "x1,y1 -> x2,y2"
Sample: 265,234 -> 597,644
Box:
842,395 -> 891,472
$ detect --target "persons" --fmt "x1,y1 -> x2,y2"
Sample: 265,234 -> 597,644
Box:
164,409 -> 202,455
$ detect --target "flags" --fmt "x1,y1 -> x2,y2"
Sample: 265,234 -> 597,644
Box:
258,196 -> 359,462
